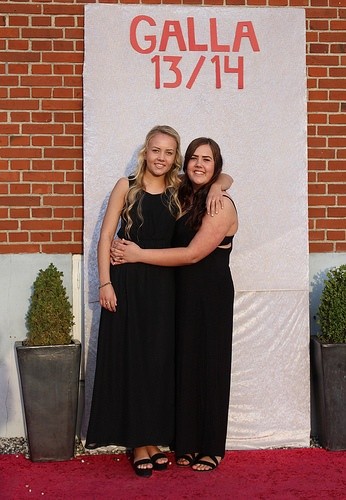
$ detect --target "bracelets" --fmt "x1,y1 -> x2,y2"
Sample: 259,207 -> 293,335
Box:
97,282 -> 112,289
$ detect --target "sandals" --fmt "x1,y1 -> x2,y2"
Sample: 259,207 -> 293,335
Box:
176,451 -> 198,467
191,454 -> 222,471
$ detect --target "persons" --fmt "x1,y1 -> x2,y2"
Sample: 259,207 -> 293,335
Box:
85,123 -> 234,477
110,135 -> 238,471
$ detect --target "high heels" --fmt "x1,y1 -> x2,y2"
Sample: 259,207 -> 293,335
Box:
150,453 -> 169,470
134,456 -> 153,476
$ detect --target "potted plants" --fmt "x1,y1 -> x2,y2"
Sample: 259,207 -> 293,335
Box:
15,261 -> 81,462
311,263 -> 346,451
